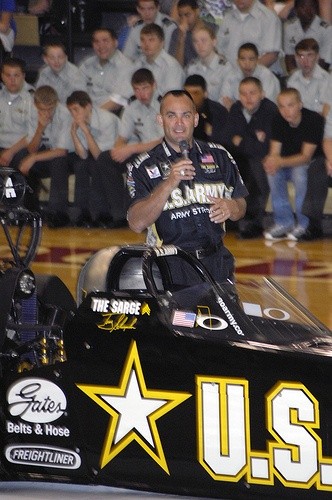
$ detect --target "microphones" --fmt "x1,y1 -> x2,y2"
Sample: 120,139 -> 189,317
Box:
179,139 -> 195,190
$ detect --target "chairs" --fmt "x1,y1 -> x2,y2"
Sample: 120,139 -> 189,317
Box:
143,243 -> 259,339
74,240 -> 172,308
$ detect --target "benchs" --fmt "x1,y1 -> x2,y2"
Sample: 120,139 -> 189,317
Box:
37,173 -> 332,216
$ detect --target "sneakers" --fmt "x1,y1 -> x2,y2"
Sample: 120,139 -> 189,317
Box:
287,225 -> 314,242
265,225 -> 297,241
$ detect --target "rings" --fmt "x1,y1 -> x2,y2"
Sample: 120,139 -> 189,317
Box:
180,170 -> 184,175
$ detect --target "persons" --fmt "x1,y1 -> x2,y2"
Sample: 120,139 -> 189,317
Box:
214,0 -> 332,74
35,23 -> 234,118
123,0 -> 219,71
219,37 -> 330,117
300,110 -> 332,240
125,90 -> 250,289
0,52 -> 39,211
9,68 -> 166,228
0,0 -> 17,82
182,74 -> 332,242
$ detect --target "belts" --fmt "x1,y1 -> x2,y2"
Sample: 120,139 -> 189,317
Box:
189,239 -> 224,259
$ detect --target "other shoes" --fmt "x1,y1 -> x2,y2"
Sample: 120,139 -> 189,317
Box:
0,207 -> 128,228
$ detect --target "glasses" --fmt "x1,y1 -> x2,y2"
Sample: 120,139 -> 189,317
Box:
298,52 -> 317,59
